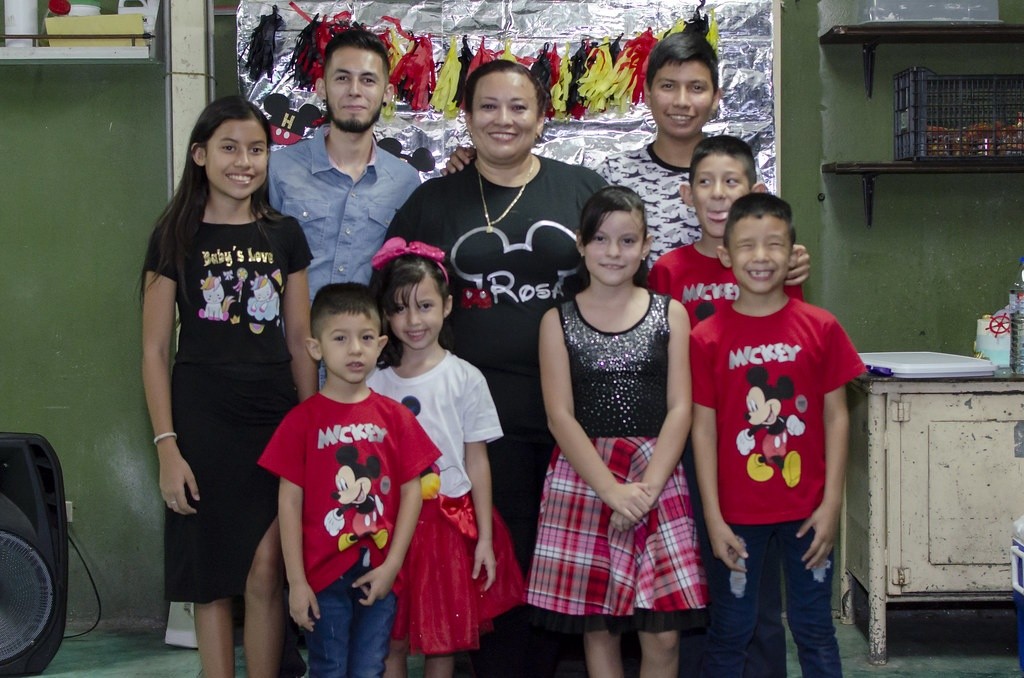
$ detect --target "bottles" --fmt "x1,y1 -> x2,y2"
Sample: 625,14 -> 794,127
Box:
1008,258 -> 1024,375
40,0 -> 71,46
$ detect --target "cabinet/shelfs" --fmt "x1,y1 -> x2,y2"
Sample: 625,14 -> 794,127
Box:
839,379 -> 1024,666
821,25 -> 1024,229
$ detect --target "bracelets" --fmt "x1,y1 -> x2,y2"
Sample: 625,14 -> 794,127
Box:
153,432 -> 177,445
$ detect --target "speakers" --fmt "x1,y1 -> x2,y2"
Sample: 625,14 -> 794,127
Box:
0,431 -> 69,678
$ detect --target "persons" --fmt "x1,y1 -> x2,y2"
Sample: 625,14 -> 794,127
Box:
688,192 -> 868,678
257,283 -> 443,678
140,96 -> 318,678
357,237 -> 527,678
440,32 -> 811,287
540,184 -> 692,678
650,135 -> 769,678
268,28 -> 423,398
370,59 -> 650,678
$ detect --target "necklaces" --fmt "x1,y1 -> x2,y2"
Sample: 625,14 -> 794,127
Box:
475,153 -> 535,233
327,150 -> 341,170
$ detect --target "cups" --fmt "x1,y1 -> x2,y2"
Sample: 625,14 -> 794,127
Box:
3,0 -> 38,47
68,4 -> 102,16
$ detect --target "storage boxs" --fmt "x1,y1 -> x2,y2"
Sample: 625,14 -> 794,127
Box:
893,66 -> 1024,162
